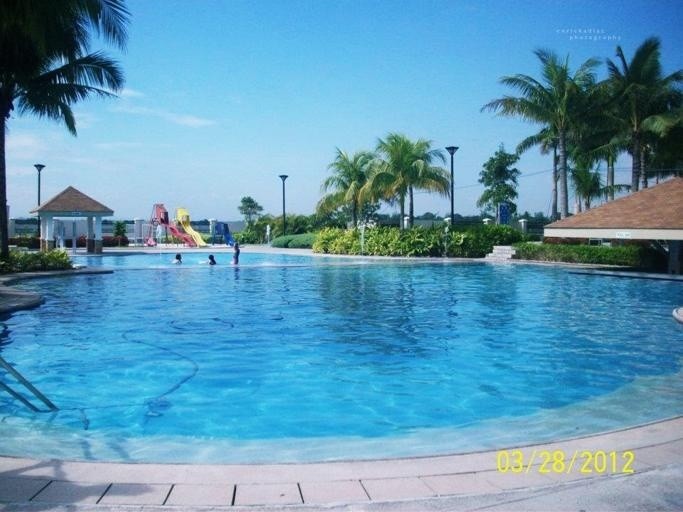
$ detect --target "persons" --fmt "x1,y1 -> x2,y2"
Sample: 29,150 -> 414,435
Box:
229,242 -> 239,266
171,253 -> 181,264
208,254 -> 215,264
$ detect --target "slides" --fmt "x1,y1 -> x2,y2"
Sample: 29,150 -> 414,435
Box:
181,221 -> 208,247
161,222 -> 197,247
224,233 -> 235,246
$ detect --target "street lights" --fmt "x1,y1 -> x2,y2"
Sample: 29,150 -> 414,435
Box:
279,173 -> 289,235
444,146 -> 459,223
33,163 -> 45,237
